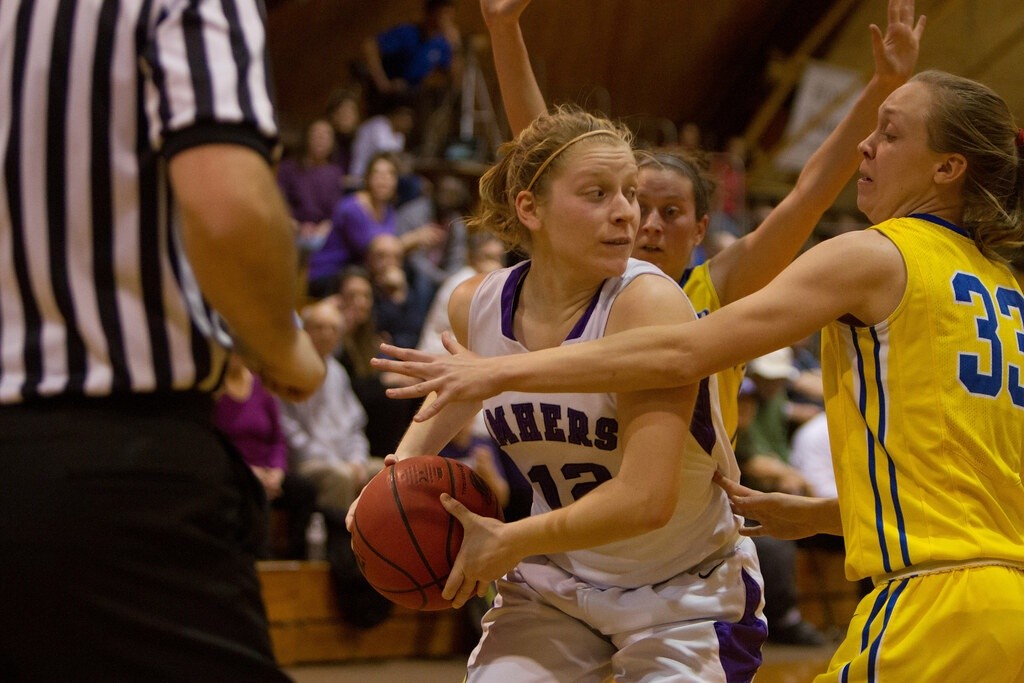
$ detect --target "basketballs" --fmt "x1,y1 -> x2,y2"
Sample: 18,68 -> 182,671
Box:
351,455 -> 505,611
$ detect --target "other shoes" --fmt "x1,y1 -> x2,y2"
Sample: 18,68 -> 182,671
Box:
768,606 -> 826,646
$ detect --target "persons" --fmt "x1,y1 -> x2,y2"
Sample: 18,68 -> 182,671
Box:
0,1 -> 327,683
213,0 -> 1024,683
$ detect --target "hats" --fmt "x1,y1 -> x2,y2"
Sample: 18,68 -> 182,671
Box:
747,347 -> 800,383
738,376 -> 757,400
